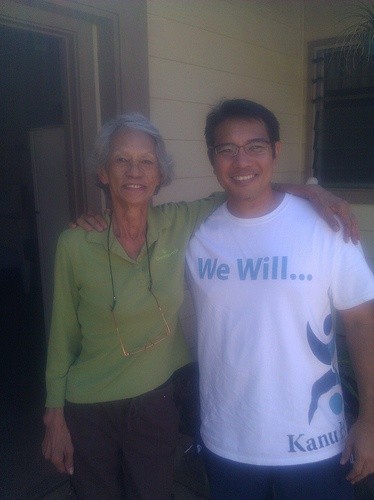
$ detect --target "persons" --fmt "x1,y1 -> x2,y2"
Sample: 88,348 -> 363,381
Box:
65,98 -> 374,500
43,111 -> 359,500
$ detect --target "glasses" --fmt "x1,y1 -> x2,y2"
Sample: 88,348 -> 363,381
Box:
210,138 -> 273,157
